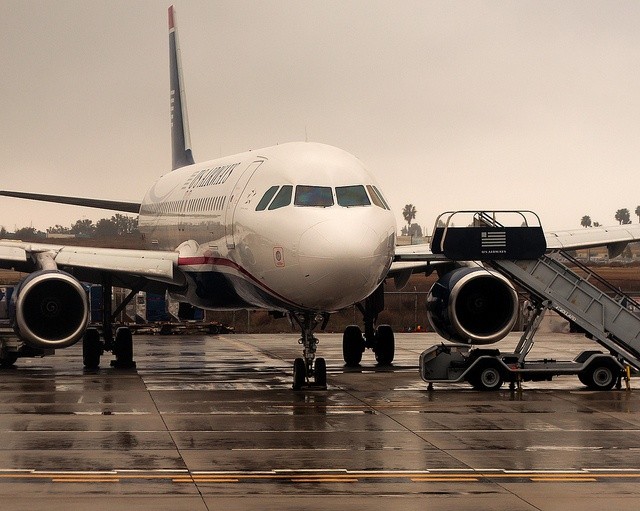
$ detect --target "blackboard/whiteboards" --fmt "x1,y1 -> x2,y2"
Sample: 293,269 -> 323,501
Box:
420,211 -> 640,391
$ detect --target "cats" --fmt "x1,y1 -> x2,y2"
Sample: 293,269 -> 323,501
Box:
0,4 -> 640,390
